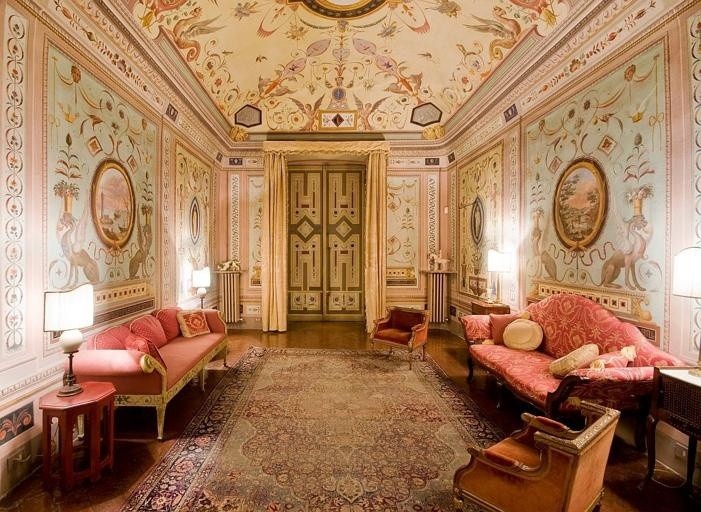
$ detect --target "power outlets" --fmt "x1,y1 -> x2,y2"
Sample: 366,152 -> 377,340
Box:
5,448 -> 26,472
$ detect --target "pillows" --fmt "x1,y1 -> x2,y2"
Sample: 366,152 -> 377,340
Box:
176,309 -> 211,338
151,307 -> 182,341
548,342 -> 599,377
575,344 -> 638,371
488,312 -> 521,345
502,318 -> 543,351
124,332 -> 167,368
121,313 -> 167,349
94,325 -> 130,349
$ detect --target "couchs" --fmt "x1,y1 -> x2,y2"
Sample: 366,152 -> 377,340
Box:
63,306 -> 228,441
457,293 -> 688,454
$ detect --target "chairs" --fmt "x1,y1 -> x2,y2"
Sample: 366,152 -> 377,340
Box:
369,305 -> 432,371
453,400 -> 622,512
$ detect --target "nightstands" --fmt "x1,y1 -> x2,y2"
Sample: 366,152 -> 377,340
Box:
469,300 -> 510,316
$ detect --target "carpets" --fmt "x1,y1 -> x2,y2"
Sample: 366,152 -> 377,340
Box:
119,345 -> 507,512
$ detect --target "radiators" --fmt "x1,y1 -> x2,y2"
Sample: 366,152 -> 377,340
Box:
215,273 -> 241,323
427,273 -> 456,324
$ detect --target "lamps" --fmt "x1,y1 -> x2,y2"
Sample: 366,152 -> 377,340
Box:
191,266 -> 211,310
45,282 -> 96,397
55,329 -> 85,397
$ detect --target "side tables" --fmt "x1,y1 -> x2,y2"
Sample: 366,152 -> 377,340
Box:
38,381 -> 117,496
639,366 -> 701,512
